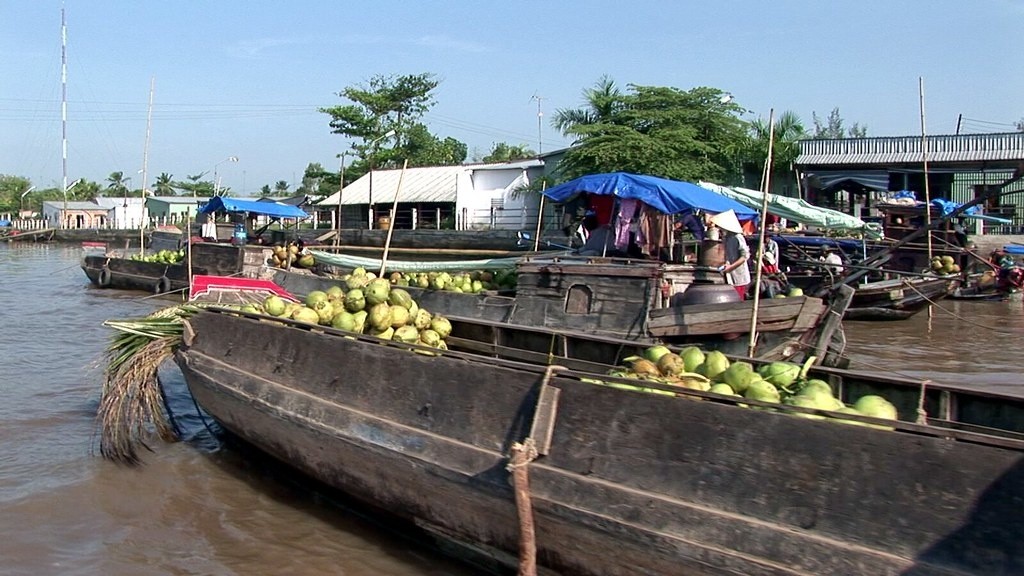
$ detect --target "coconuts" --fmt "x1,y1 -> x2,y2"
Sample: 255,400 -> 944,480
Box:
932,256 -> 961,275
270,245 -> 315,268
222,267 -> 518,354
579,346 -> 898,432
773,288 -> 802,297
131,249 -> 185,264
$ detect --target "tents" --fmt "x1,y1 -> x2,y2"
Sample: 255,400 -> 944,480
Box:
542,170 -> 755,255
198,196 -> 306,229
697,179 -> 882,283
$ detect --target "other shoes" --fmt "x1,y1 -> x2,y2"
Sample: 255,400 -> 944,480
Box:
724,333 -> 740,340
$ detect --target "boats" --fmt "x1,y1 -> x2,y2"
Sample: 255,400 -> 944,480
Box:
80,231 -> 289,295
0,225 -> 57,242
507,170 -> 979,344
171,271 -> 1024,575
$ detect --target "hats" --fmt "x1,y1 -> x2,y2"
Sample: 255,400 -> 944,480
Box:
821,245 -> 829,251
710,209 -> 743,233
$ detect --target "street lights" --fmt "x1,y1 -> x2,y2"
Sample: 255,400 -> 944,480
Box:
368,130 -> 397,229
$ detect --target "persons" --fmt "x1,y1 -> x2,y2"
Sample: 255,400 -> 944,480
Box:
756,232 -> 779,274
711,209 -> 750,302
823,245 -> 844,273
245,212 -> 257,239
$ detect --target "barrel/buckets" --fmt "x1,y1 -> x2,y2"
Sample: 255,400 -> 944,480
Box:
697,239 -> 725,268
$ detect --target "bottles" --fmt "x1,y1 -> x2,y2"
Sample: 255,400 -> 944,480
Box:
234,223 -> 246,244
